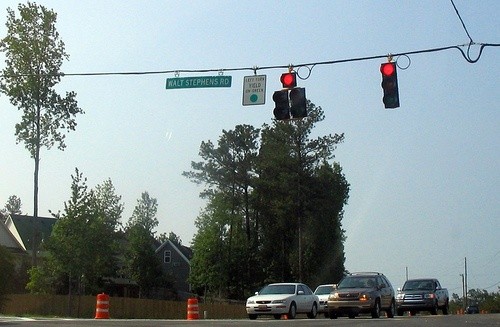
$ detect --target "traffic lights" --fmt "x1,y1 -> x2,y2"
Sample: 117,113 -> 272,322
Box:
281,72 -> 297,88
289,87 -> 307,117
272,87 -> 290,120
381,61 -> 399,109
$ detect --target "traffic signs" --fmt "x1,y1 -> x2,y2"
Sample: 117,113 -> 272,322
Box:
242,74 -> 266,106
165,76 -> 231,89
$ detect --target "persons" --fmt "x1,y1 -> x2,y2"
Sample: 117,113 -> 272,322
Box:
367,279 -> 374,287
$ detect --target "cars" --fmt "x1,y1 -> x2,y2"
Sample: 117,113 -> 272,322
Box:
314,284 -> 338,317
245,282 -> 320,321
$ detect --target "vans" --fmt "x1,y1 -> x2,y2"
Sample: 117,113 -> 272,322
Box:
464,305 -> 479,315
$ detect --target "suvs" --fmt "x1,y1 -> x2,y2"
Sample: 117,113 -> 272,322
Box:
327,272 -> 396,318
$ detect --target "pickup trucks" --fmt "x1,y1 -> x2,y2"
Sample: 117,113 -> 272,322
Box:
396,279 -> 450,316
